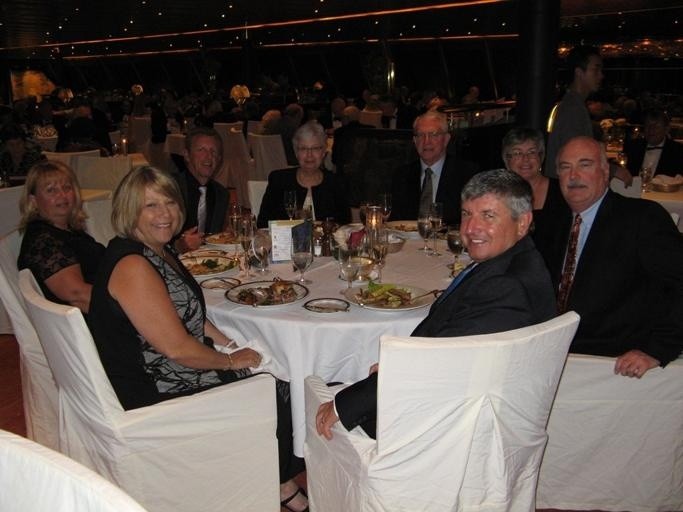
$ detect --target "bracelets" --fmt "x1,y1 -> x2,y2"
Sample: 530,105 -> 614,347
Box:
223,352 -> 232,371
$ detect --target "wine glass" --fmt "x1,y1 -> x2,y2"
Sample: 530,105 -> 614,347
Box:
289,236 -> 315,287
229,199 -> 272,281
339,204 -> 391,295
446,229 -> 466,268
418,201 -> 444,259
284,188 -> 312,221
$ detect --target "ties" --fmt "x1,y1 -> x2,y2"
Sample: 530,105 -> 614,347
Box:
197,186 -> 208,237
418,168 -> 434,220
555,213 -> 582,317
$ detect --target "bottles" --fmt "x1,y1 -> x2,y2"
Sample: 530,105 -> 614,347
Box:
315,217 -> 334,257
640,167 -> 653,192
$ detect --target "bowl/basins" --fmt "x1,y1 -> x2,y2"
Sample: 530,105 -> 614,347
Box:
651,181 -> 681,193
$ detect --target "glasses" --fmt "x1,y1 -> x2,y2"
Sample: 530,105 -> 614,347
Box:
298,147 -> 322,152
509,149 -> 540,160
414,132 -> 446,137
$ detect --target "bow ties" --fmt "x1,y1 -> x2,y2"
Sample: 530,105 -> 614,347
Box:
647,145 -> 662,151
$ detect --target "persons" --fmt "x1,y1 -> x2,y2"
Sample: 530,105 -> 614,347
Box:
0,46 -> 681,185
87,162 -> 308,512
539,133 -> 683,381
251,118 -> 353,226
385,107 -> 468,223
170,123 -> 233,251
496,123 -> 573,242
15,160 -> 110,321
315,167 -> 559,443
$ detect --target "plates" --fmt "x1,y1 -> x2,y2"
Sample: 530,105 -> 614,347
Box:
302,298 -> 350,314
222,280 -> 309,307
449,269 -> 465,279
202,232 -> 243,246
341,283 -> 435,312
178,250 -> 235,277
200,277 -> 241,291
383,220 -> 419,239
